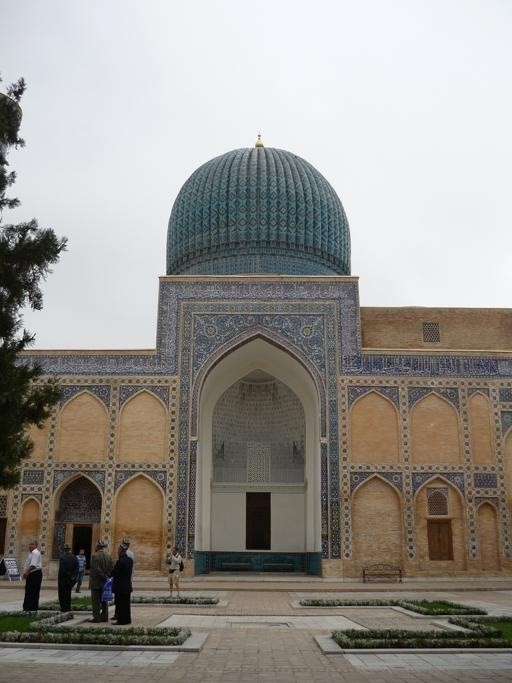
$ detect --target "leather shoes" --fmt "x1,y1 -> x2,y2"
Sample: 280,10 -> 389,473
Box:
89,617 -> 124,624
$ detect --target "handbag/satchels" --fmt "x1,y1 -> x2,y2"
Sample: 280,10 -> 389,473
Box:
177,555 -> 184,571
169,569 -> 177,574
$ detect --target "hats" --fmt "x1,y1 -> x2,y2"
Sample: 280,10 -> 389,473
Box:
120,539 -> 130,550
63,543 -> 73,550
97,539 -> 108,548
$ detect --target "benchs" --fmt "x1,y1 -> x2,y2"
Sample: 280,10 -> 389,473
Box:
363,564 -> 402,584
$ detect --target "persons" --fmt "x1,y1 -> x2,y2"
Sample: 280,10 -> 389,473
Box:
165,546 -> 182,599
110,544 -> 133,626
71,549 -> 86,593
87,539 -> 114,623
58,543 -> 80,612
21,540 -> 43,612
109,538 -> 135,606
